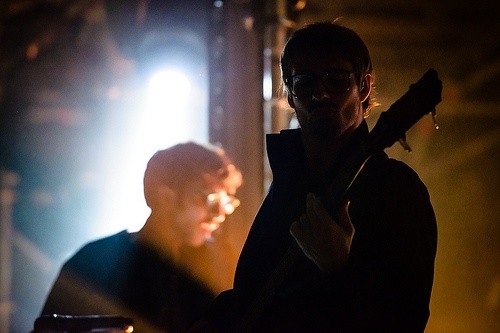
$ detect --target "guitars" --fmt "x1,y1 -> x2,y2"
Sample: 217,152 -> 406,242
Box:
204,67 -> 444,332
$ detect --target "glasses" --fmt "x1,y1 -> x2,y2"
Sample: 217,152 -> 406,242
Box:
194,189 -> 241,215
286,65 -> 360,99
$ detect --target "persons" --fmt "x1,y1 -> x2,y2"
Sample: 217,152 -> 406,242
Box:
27,138 -> 246,333
193,16 -> 441,333
